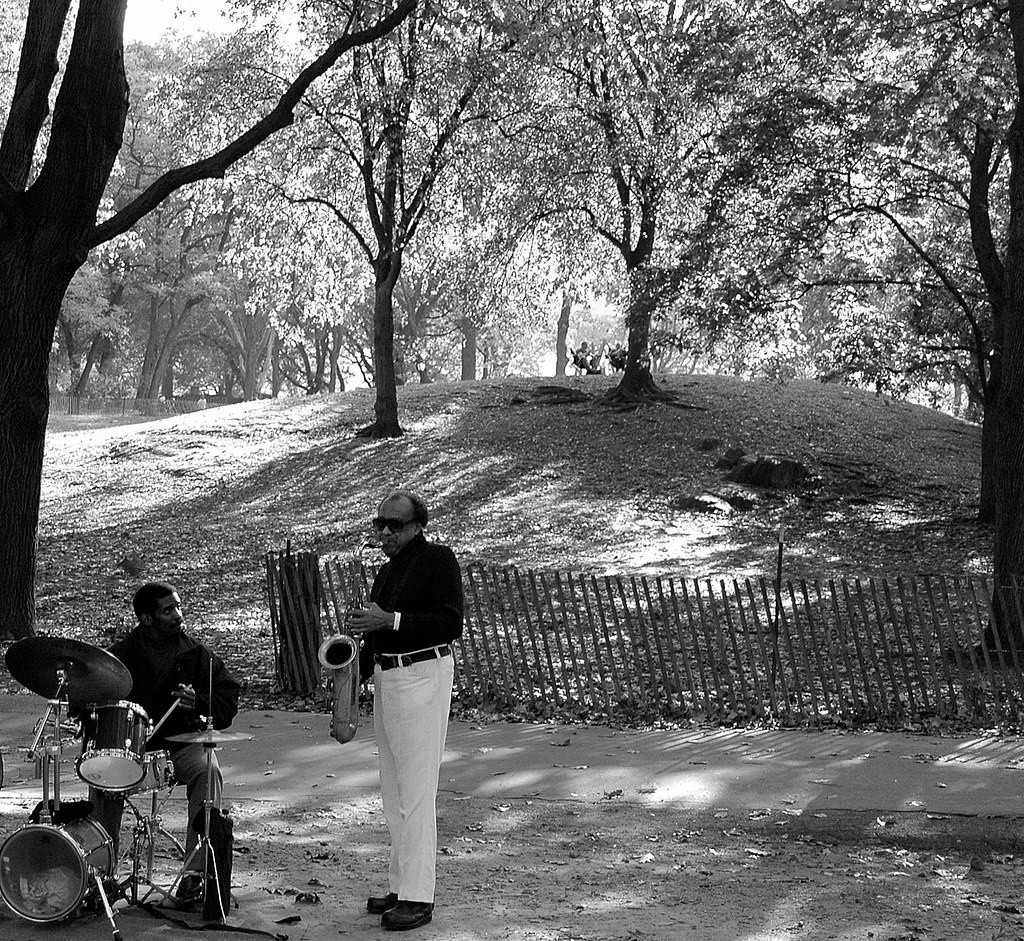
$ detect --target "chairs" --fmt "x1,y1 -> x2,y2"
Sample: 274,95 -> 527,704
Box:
569,348 -> 586,375
603,347 -> 617,373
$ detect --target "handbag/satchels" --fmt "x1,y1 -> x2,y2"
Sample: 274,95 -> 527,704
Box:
191,807 -> 235,921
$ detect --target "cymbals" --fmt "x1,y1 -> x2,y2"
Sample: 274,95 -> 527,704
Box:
165,730 -> 249,745
5,636 -> 133,706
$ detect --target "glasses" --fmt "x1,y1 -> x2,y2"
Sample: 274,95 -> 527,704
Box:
372,517 -> 418,533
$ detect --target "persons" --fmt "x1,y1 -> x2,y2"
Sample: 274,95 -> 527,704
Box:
92,582 -> 241,905
576,342 -> 601,375
345,491 -> 464,930
609,343 -> 629,368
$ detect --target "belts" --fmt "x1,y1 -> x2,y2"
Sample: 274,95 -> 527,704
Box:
378,645 -> 451,671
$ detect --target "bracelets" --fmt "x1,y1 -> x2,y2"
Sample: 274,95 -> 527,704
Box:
394,612 -> 401,631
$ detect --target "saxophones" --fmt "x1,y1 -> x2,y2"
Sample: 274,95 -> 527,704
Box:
317,538 -> 387,746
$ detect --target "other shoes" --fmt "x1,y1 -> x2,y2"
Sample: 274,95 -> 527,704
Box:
176,875 -> 203,899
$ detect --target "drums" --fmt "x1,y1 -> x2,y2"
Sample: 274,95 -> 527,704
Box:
121,750 -> 171,794
0,814 -> 116,924
73,696 -> 152,791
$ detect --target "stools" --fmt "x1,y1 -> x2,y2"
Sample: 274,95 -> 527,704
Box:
144,781 -> 185,894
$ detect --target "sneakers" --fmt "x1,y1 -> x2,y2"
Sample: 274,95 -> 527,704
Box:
381,901 -> 432,931
367,893 -> 398,914
